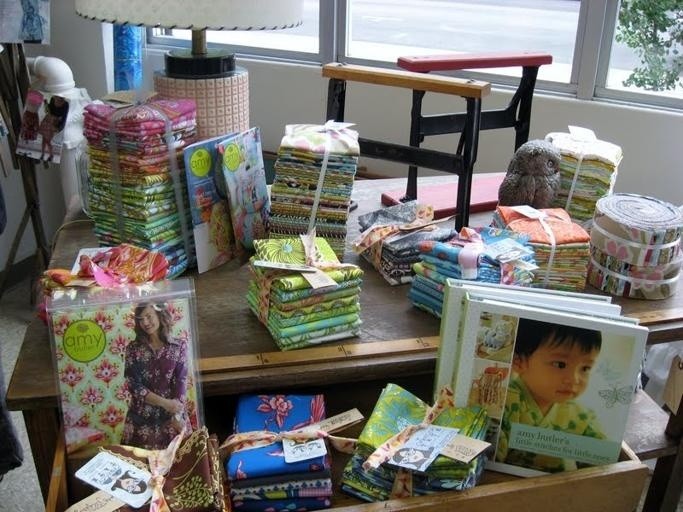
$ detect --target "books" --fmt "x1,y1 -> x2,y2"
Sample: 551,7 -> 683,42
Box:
434,277 -> 648,479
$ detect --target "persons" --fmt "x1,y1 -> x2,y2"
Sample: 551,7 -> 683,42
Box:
112,471 -> 146,495
120,302 -> 193,447
39,95 -> 69,161
21,91 -> 43,141
395,448 -> 431,469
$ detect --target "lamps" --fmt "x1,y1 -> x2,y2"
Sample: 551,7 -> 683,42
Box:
72,0 -> 305,140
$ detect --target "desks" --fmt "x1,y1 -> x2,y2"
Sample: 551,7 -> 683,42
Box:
4,162 -> 683,512
